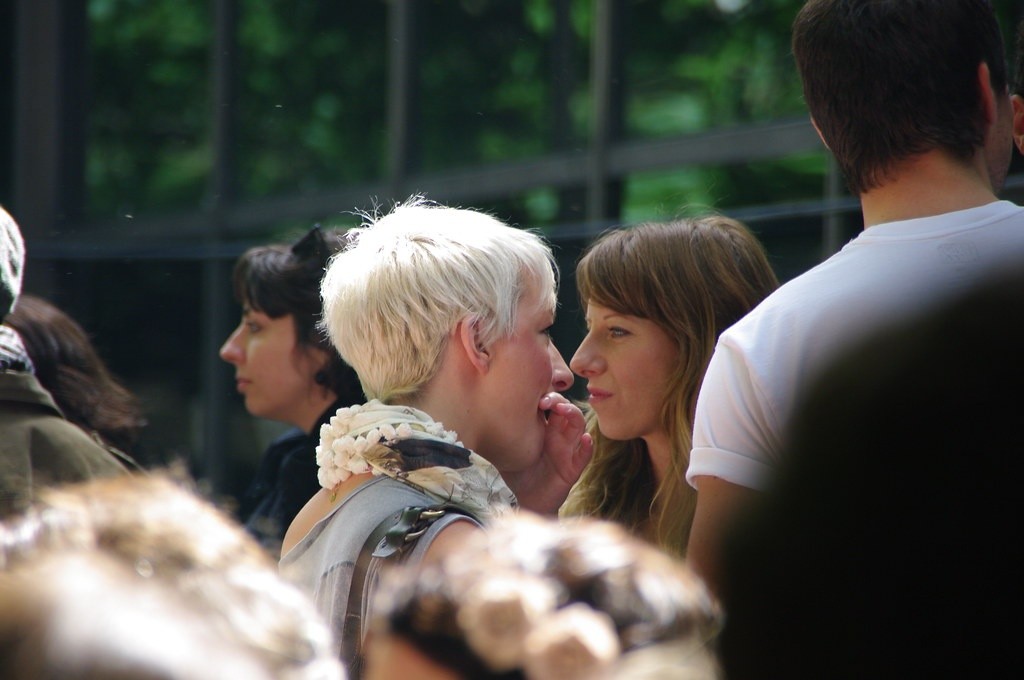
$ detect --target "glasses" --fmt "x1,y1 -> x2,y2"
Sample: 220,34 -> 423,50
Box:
290,222 -> 330,259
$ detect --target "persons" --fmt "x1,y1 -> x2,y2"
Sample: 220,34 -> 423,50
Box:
557,216 -> 780,561
360,517 -> 723,680
0,206 -> 341,680
686,0 -> 1024,680
282,198 -> 594,667
223,224 -> 362,551
